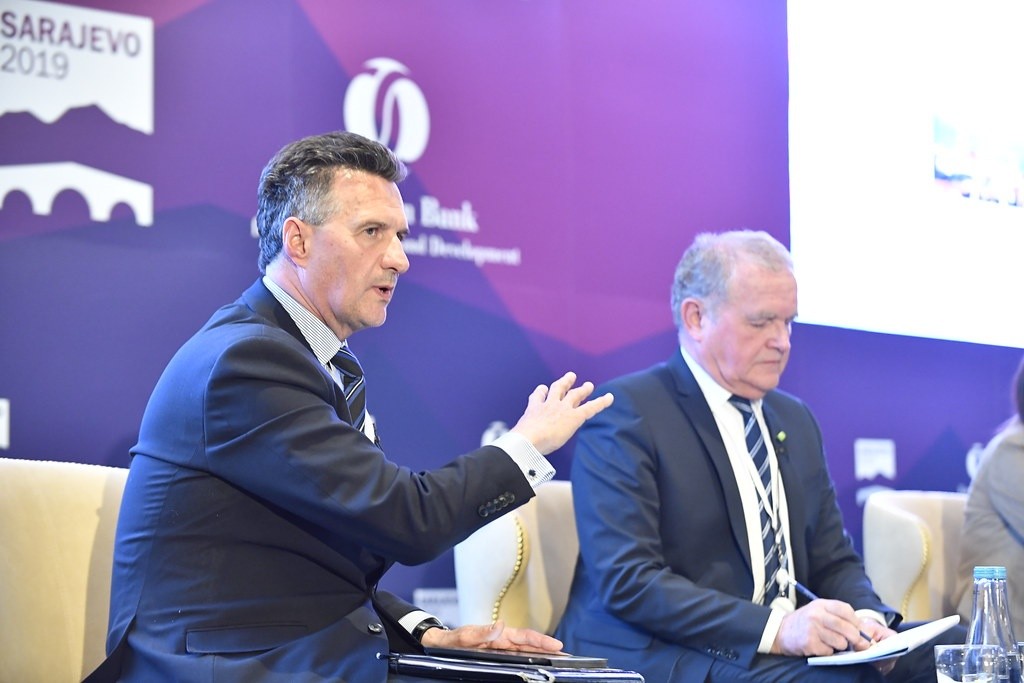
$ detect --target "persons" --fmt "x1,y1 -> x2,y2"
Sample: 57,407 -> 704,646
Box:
110,132 -> 617,681
576,231 -> 967,683
954,353 -> 1024,645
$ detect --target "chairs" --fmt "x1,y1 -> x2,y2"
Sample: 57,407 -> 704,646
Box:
452,479 -> 580,637
0,457 -> 130,683
862,490 -> 967,623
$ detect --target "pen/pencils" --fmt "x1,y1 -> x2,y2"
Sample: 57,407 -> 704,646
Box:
786,575 -> 878,647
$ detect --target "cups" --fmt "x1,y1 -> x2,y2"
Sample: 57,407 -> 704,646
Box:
933,644 -> 1001,683
1014,641 -> 1024,683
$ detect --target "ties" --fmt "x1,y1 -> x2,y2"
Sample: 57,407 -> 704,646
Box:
328,346 -> 366,433
727,393 -> 790,607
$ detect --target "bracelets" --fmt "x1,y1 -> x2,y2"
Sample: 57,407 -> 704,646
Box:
412,622 -> 450,643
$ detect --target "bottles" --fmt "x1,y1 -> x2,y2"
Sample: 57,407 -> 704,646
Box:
961,565 -> 1021,683
